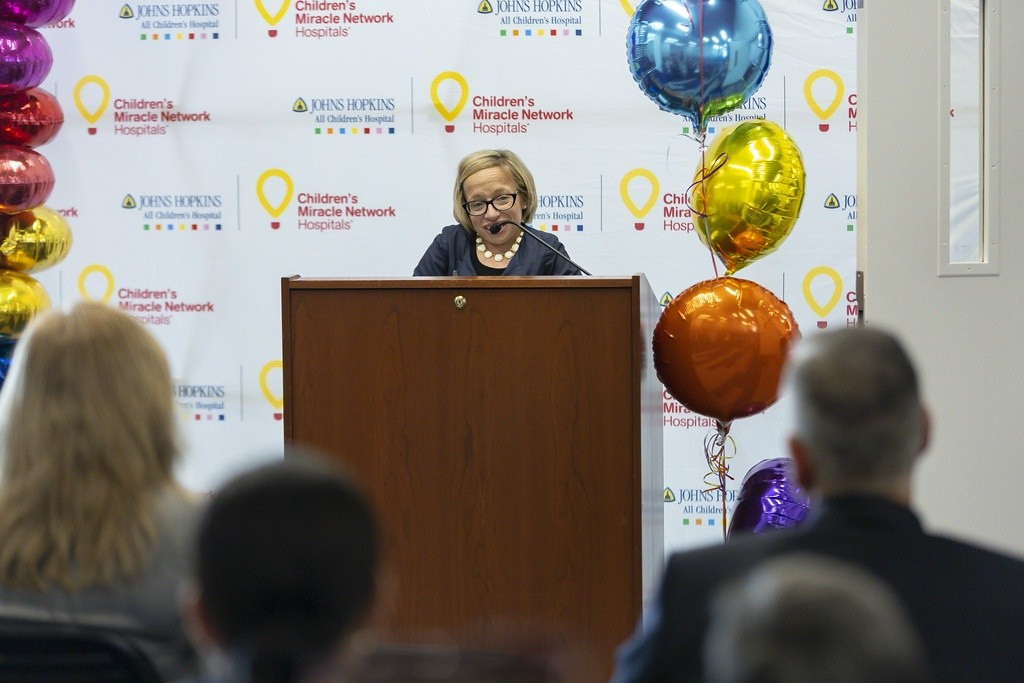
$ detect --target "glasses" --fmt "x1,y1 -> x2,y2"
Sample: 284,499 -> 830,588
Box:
462,190 -> 519,216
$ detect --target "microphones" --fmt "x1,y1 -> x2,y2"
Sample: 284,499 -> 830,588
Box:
489,221 -> 593,275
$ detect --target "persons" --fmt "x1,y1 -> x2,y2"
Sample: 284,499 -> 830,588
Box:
0,301 -> 246,683
187,460 -> 378,683
412,149 -> 583,276
611,326 -> 1024,683
699,551 -> 925,683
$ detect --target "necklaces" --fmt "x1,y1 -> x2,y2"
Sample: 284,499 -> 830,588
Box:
476,231 -> 524,261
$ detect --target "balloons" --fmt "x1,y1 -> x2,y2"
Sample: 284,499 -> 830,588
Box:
690,119 -> 806,275
626,0 -> 774,132
0,0 -> 75,389
725,458 -> 811,545
652,277 -> 802,443
